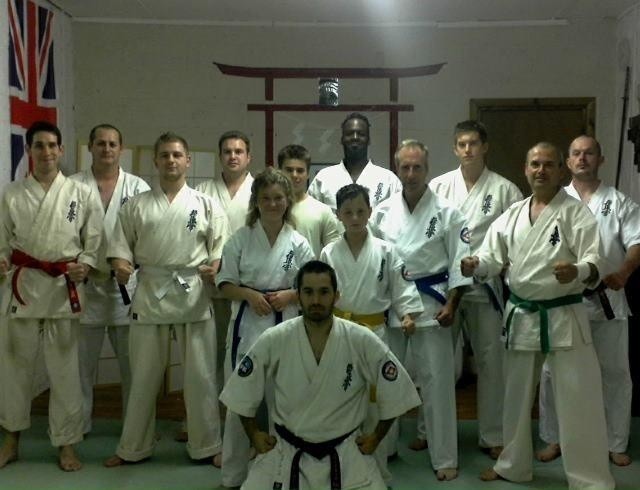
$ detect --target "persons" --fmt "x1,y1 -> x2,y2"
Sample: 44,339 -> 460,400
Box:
533,133 -> 639,468
172,127 -> 258,444
214,164 -> 319,490
276,143 -> 342,261
218,260 -> 421,490
318,184 -> 427,490
65,121 -> 165,445
368,136 -> 478,482
2,119 -> 105,470
406,116 -> 525,460
104,135 -> 230,470
460,140 -> 618,490
305,111 -> 406,212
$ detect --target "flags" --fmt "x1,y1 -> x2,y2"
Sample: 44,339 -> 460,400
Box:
5,0 -> 63,187
